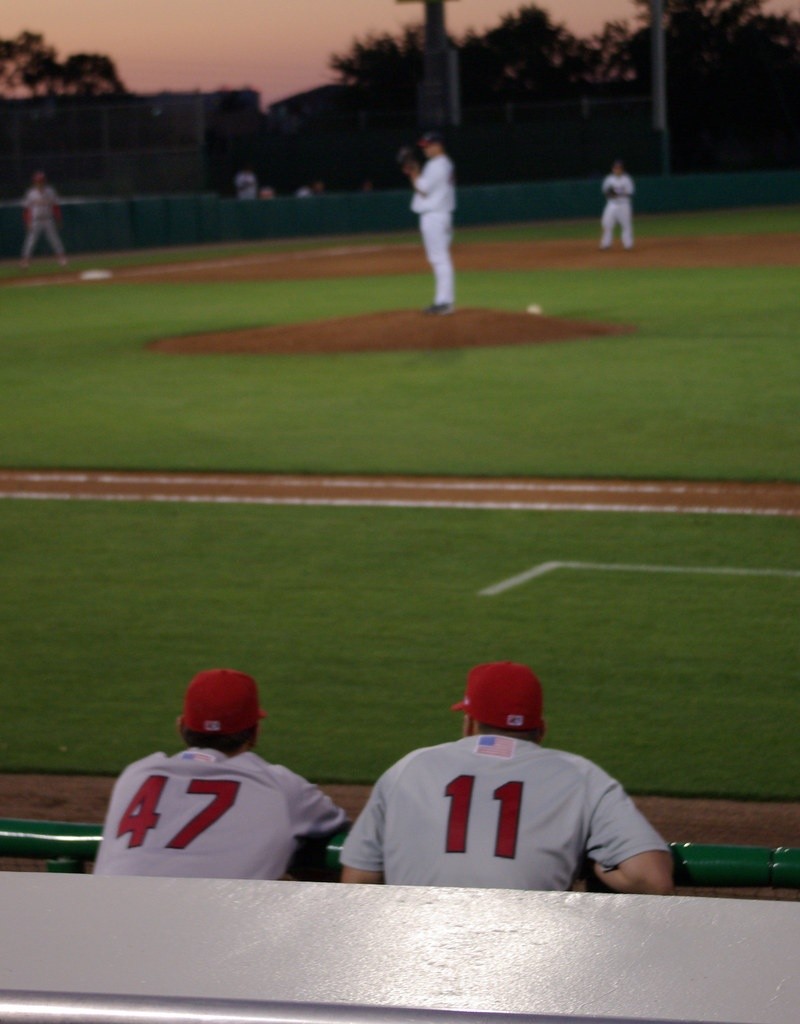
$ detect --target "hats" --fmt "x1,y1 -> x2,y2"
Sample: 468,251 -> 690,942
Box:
182,668 -> 268,733
450,661 -> 545,730
417,131 -> 447,146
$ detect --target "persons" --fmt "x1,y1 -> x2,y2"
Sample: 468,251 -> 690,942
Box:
339,662 -> 675,896
235,167 -> 256,201
19,171 -> 68,269
599,162 -> 634,251
93,670 -> 351,881
403,134 -> 457,314
295,181 -> 324,199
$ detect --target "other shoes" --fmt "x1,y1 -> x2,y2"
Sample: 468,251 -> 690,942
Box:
421,304 -> 455,316
